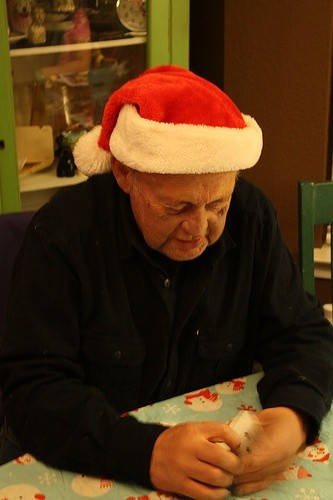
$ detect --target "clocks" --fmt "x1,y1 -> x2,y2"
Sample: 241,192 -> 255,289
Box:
116,1 -> 148,37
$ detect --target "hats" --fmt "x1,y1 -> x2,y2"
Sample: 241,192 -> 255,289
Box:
72,63 -> 264,178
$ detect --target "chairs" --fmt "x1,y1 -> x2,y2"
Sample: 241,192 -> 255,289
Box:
298,180 -> 332,297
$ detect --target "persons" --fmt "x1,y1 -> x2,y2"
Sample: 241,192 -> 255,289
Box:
0,67 -> 333,500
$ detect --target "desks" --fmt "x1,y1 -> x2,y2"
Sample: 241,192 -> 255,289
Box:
1,369 -> 332,500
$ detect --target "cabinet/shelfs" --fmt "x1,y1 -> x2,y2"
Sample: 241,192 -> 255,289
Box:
0,0 -> 190,214
